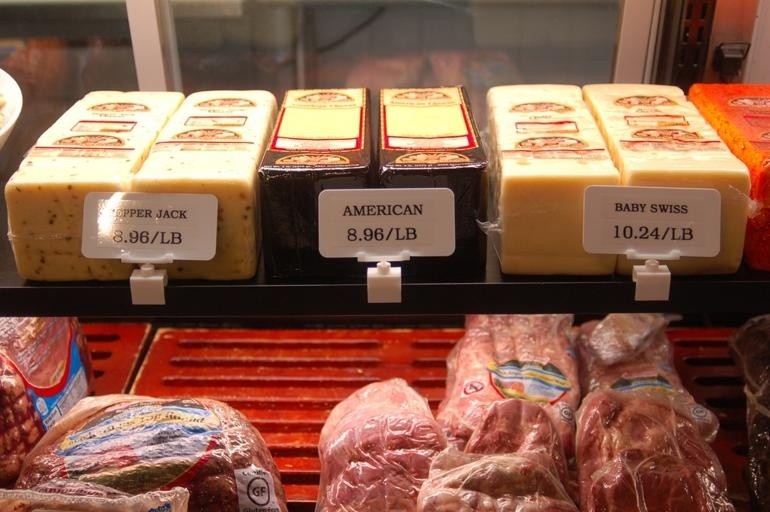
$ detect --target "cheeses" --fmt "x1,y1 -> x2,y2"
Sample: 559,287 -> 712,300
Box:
5,83 -> 769,282
